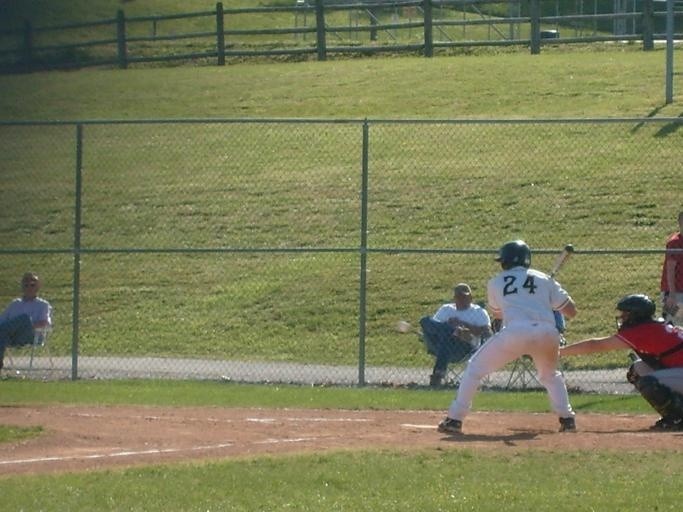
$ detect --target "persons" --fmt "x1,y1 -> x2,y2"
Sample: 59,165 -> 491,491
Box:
558,293 -> 682,431
437,239 -> 577,435
0,272 -> 52,375
660,212 -> 683,328
418,282 -> 492,387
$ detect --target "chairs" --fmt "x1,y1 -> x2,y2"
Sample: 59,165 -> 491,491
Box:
422,331 -> 567,392
4,306 -> 55,380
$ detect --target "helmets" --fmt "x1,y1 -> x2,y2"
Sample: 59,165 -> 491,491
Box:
493,240 -> 531,268
615,294 -> 655,320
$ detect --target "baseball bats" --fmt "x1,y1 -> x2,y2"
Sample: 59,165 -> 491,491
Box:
548,245 -> 573,278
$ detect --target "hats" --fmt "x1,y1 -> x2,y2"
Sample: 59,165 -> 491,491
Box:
454,283 -> 471,296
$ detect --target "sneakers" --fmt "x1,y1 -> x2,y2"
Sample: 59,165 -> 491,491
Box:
429,374 -> 440,386
437,417 -> 462,435
650,418 -> 682,431
558,417 -> 576,432
